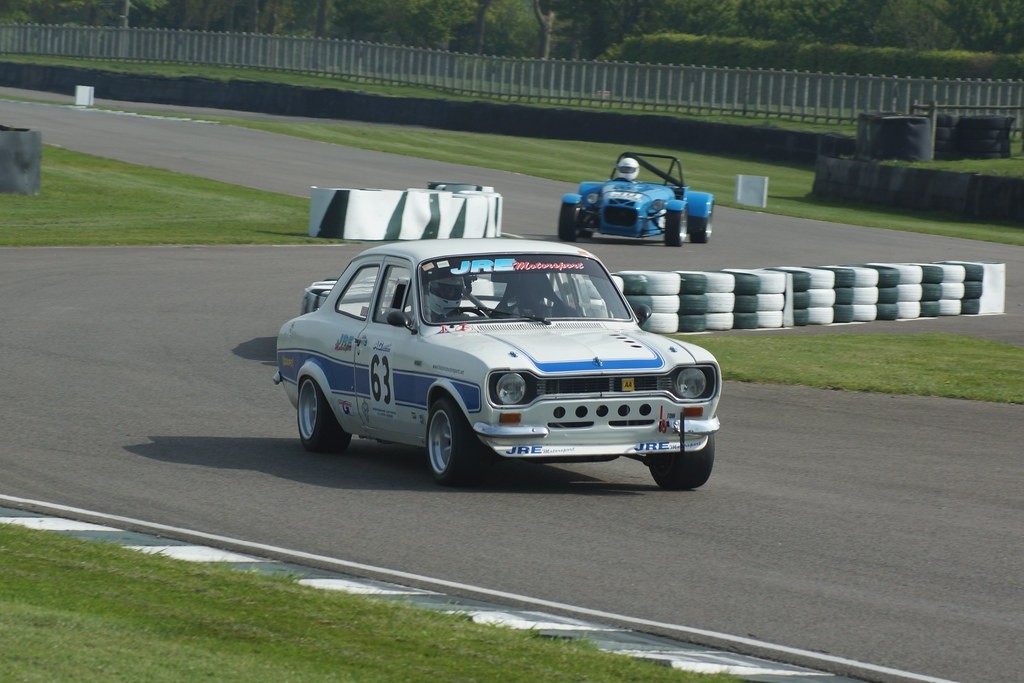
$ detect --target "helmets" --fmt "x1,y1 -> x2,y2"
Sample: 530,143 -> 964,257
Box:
427,275 -> 465,314
618,158 -> 640,180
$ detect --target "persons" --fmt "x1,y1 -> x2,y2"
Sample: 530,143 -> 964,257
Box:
616,158 -> 639,183
428,275 -> 464,322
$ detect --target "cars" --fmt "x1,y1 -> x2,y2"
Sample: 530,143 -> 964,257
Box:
270,235 -> 723,491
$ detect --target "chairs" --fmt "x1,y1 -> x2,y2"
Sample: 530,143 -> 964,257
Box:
492,270 -> 578,319
